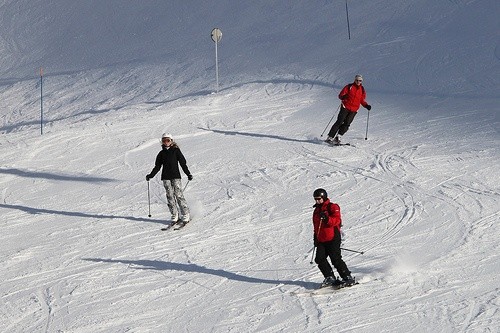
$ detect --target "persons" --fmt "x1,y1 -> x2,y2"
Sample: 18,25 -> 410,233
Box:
145,132 -> 194,225
325,74 -> 371,143
312,189 -> 356,290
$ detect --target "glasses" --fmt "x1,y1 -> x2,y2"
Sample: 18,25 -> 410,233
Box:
160,139 -> 171,144
314,197 -> 322,200
357,80 -> 362,83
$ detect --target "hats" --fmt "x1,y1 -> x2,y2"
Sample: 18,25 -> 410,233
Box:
355,74 -> 363,80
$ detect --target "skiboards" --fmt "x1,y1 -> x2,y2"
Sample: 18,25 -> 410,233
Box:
319,140 -> 351,146
160,221 -> 186,231
289,277 -> 380,295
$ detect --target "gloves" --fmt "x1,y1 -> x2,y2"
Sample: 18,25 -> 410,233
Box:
188,174 -> 192,180
367,105 -> 371,110
313,239 -> 318,247
146,174 -> 152,181
320,214 -> 328,222
342,95 -> 346,99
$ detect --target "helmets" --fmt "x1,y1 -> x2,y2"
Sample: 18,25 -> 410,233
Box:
162,132 -> 173,140
313,189 -> 327,200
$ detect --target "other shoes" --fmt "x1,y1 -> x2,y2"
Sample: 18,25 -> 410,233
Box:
339,275 -> 354,288
171,214 -> 179,222
334,135 -> 341,142
182,213 -> 190,222
321,276 -> 336,287
326,136 -> 332,142
